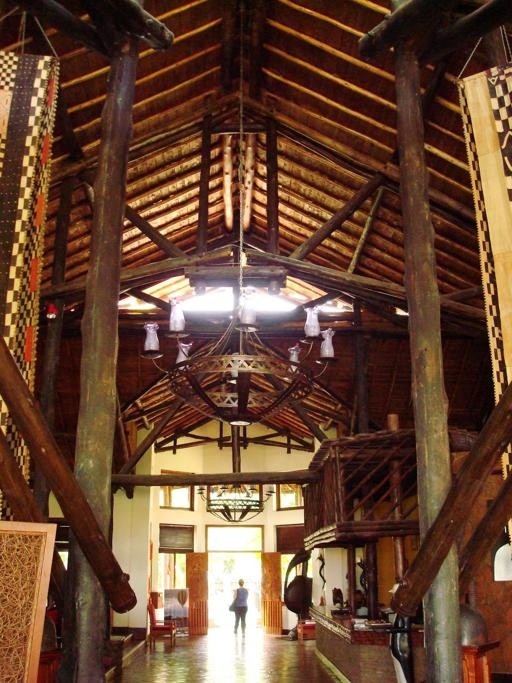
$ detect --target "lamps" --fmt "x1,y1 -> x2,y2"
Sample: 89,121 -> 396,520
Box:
131,289 -> 344,429
193,481 -> 278,524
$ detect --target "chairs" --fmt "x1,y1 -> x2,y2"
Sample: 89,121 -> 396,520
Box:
143,601 -> 178,651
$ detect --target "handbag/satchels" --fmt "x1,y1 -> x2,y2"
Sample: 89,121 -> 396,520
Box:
229,602 -> 236,611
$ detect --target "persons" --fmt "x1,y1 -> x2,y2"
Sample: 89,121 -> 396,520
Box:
232,578 -> 249,640
